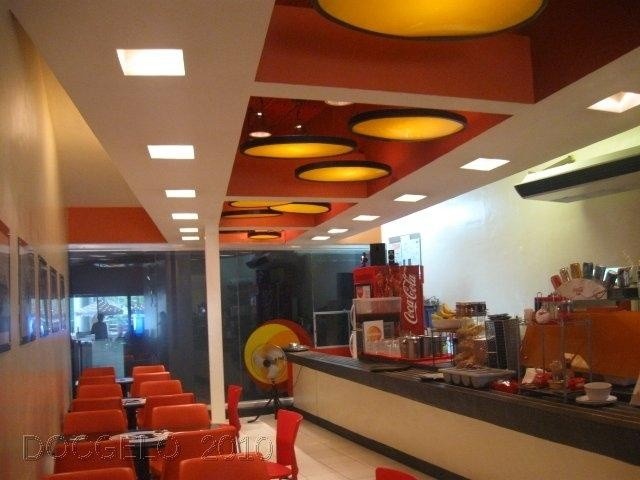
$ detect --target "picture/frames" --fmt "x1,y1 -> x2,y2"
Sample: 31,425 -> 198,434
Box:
0,221 -> 66,352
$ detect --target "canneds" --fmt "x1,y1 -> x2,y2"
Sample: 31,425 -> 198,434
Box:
551,262 -> 629,289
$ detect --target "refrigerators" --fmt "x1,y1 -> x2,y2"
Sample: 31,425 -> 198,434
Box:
348,265 -> 424,359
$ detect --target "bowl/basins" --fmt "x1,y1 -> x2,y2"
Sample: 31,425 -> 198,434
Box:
585,381 -> 612,400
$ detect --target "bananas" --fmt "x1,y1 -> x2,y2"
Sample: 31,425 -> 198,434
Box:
431,304 -> 456,320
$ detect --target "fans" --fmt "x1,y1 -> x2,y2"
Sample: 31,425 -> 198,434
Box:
246,342 -> 289,423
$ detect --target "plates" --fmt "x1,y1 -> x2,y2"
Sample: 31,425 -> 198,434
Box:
575,396 -> 618,406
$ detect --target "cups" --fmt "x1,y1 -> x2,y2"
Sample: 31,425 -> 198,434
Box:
366,336 -> 403,358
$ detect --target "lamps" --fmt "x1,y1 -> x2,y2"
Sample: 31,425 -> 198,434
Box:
219,107 -> 467,239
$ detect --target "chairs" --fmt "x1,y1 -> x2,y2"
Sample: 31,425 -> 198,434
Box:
38,363 -> 305,480
375,465 -> 421,480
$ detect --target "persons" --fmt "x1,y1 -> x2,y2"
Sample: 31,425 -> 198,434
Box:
90,313 -> 108,339
156,311 -> 168,337
